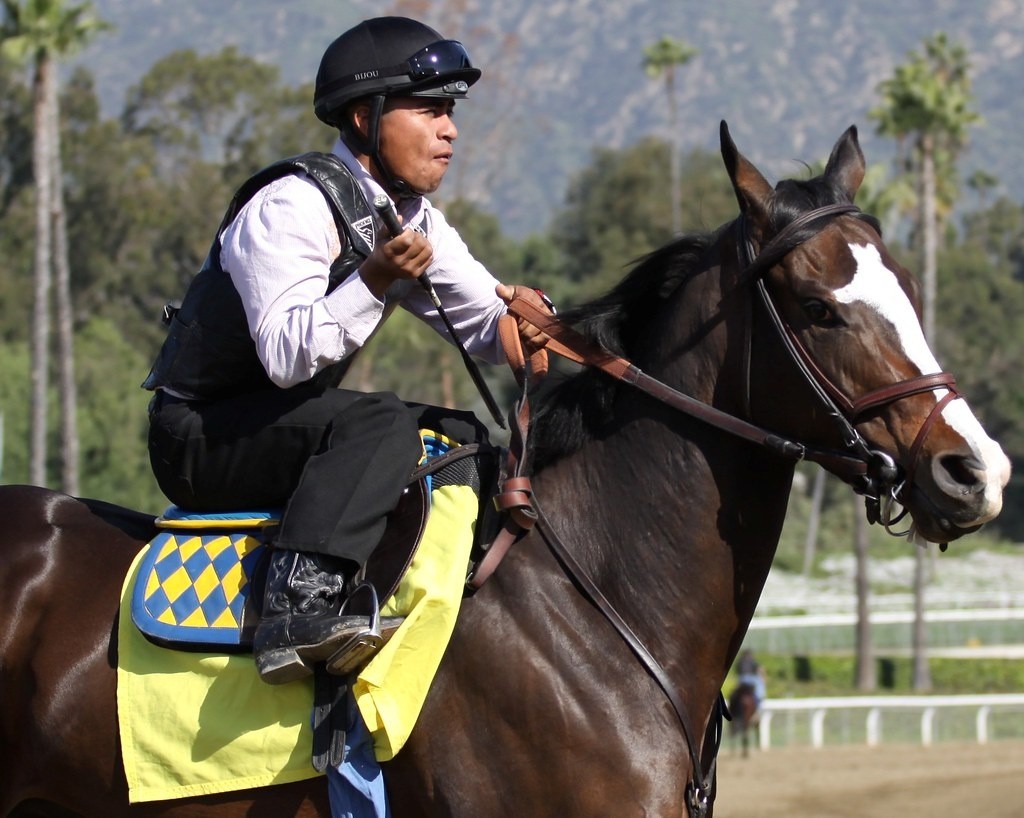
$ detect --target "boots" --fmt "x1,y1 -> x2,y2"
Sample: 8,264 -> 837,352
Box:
253,550 -> 408,686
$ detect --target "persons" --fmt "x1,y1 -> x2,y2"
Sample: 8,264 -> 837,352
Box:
730,650 -> 767,756
144,16 -> 560,684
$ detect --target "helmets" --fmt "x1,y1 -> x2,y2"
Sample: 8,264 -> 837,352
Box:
312,15 -> 483,130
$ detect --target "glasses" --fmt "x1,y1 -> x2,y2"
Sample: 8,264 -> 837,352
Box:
408,38 -> 472,78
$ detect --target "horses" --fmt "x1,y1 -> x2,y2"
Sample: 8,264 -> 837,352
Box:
0,120 -> 1012,818
